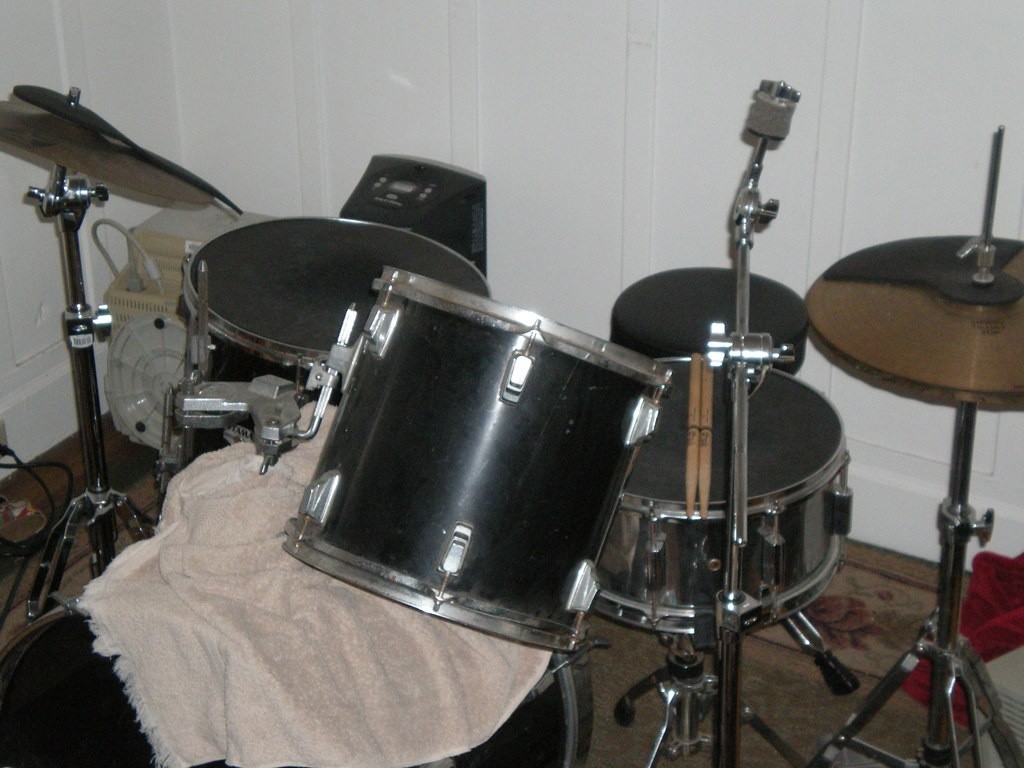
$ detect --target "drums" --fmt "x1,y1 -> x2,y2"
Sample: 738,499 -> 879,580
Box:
589,356 -> 850,642
281,263 -> 675,652
1,601 -> 595,768
180,216 -> 494,478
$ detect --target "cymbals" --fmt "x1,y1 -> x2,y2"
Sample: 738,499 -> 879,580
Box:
0,102 -> 216,203
803,235 -> 1024,412
